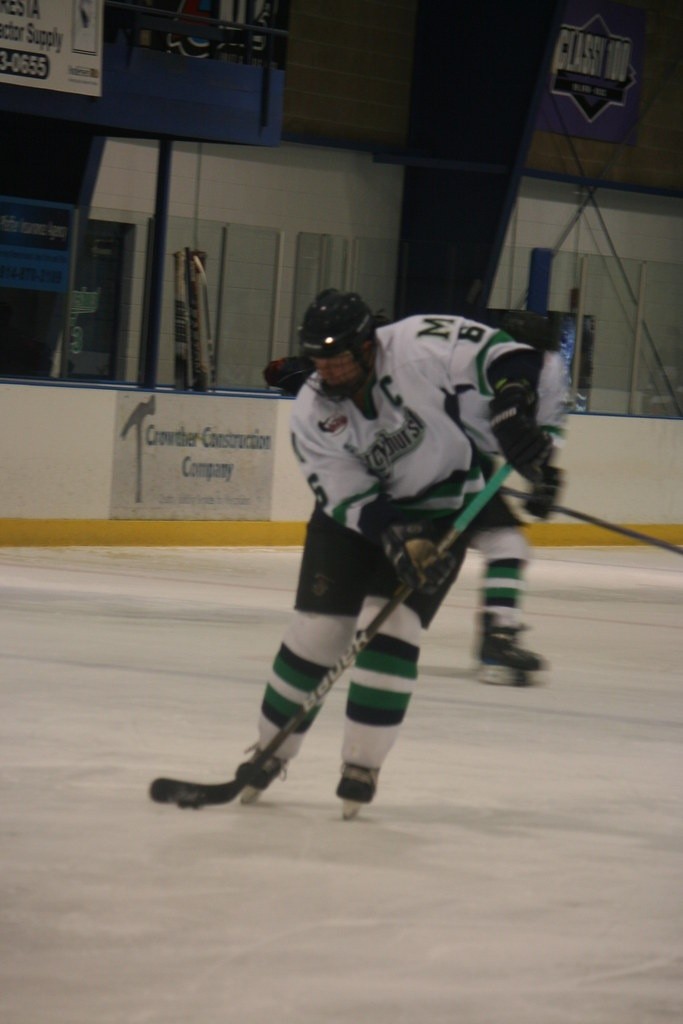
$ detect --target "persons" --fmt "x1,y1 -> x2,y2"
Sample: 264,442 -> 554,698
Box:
460,312 -> 571,687
237,287 -> 545,821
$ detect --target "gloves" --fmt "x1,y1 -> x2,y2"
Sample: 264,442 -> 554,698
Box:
488,395 -> 553,479
264,356 -> 319,397
372,519 -> 455,594
523,466 -> 561,518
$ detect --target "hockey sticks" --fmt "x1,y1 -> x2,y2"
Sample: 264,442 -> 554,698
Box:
151,461 -> 512,808
496,484 -> 683,554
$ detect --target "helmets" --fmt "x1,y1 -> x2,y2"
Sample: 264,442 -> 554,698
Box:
298,288 -> 377,396
499,308 -> 547,348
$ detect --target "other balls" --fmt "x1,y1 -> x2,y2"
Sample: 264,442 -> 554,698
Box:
177,797 -> 204,811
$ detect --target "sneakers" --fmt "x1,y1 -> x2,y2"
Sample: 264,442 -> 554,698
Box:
475,612 -> 540,685
235,743 -> 288,804
335,761 -> 379,819
